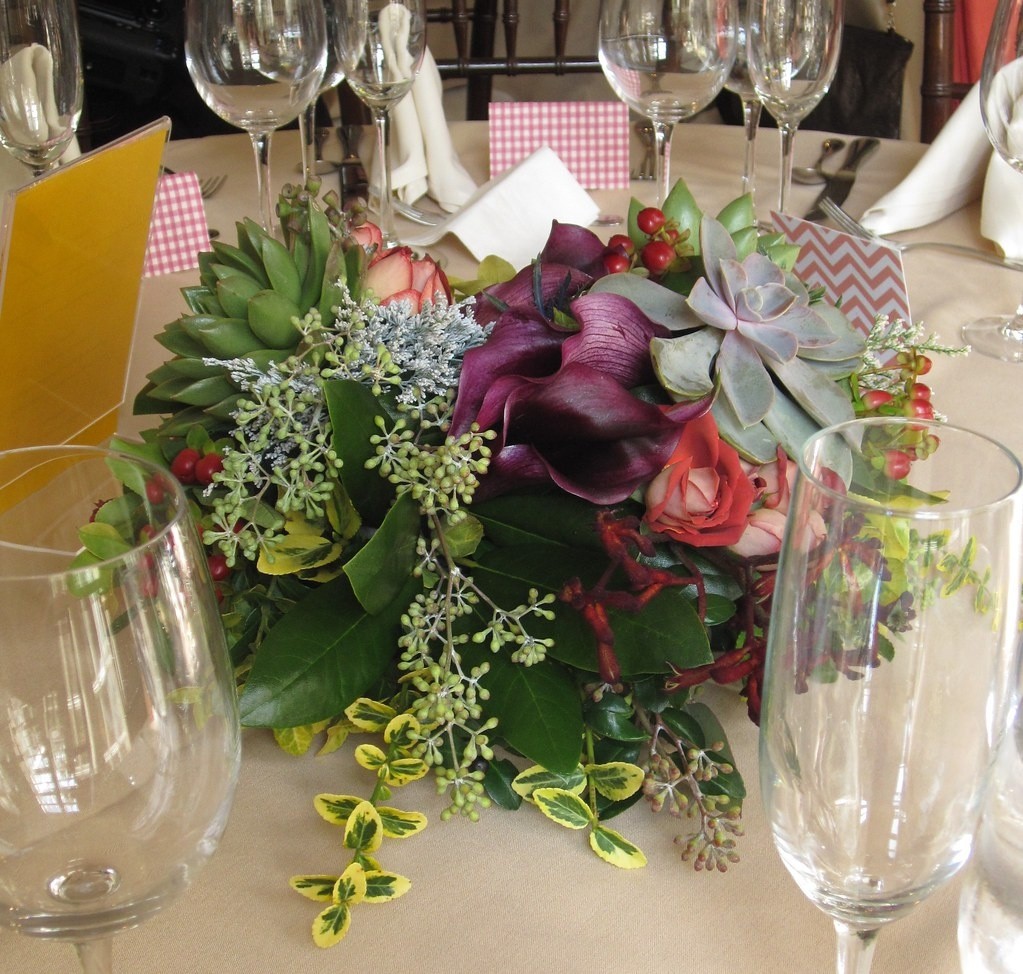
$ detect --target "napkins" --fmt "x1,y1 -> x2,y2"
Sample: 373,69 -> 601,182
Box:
367,2 -> 477,213
857,56 -> 1022,269
0,45 -> 80,167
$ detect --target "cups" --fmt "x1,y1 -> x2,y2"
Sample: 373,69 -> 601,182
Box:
957,710 -> 1021,974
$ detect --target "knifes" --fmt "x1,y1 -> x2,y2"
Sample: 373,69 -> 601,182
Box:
803,138 -> 880,222
334,123 -> 370,212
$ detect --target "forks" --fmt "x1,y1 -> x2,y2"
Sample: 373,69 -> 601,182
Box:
367,186 -> 624,226
631,123 -> 658,178
161,165 -> 227,198
818,196 -> 1023,272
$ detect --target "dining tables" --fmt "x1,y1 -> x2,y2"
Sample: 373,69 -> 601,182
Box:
0,123 -> 1023,974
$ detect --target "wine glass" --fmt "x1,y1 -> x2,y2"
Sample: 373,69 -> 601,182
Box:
0,0 -> 83,184
0,445 -> 240,974
756,413 -> 1021,974
597,0 -> 845,238
180,0 -> 447,268
963,0 -> 1023,363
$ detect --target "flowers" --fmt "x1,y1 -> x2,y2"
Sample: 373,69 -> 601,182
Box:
39,172 -> 990,950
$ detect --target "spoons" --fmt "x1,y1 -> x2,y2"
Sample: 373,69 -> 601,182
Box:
791,139 -> 844,184
298,128 -> 336,175
